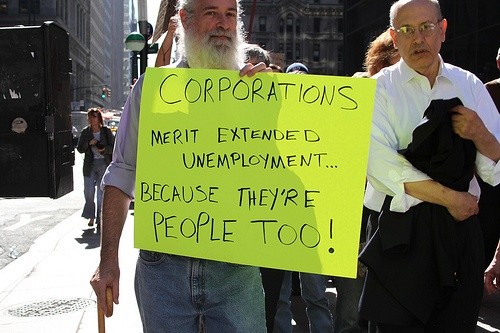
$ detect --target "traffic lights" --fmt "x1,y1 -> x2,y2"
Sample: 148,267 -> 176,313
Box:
107,88 -> 111,98
102,89 -> 106,100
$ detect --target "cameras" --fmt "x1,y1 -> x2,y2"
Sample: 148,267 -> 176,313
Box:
95,140 -> 104,149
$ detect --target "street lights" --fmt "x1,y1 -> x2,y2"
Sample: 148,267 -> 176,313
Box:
125,31 -> 145,91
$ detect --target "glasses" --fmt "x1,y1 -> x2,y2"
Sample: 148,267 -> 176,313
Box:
393,18 -> 441,37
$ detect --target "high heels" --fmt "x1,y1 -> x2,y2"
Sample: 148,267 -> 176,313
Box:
96,225 -> 101,234
88,219 -> 94,227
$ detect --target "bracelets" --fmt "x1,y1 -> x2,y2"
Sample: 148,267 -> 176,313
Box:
161,45 -> 173,64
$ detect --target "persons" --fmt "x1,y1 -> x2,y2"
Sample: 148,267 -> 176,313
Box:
358,0 -> 500,333
90,0 -> 273,333
263,29 -> 500,333
155,15 -> 271,70
77,108 -> 115,226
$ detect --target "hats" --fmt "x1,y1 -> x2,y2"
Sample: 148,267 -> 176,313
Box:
286,63 -> 308,72
